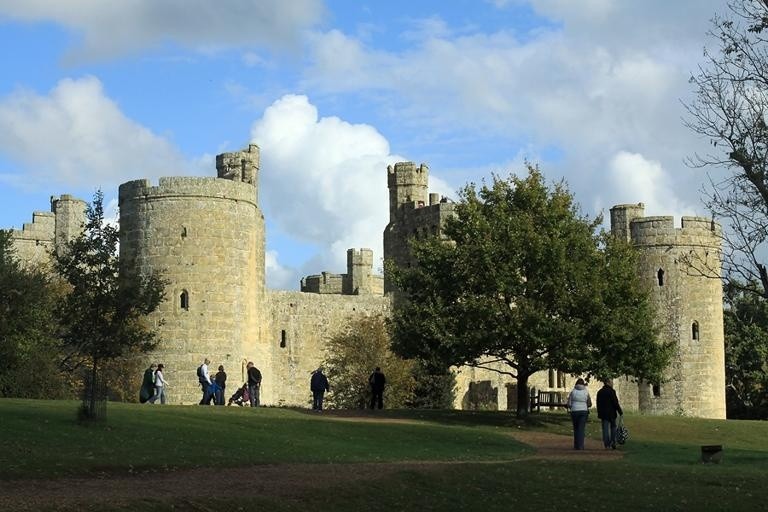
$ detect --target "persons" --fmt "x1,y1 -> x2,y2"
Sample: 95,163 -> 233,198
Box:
142,363 -> 157,403
144,363 -> 170,404
247,361 -> 262,407
596,375 -> 624,450
368,366 -> 386,413
310,366 -> 330,413
198,358 -> 212,406
566,377 -> 592,451
214,365 -> 226,405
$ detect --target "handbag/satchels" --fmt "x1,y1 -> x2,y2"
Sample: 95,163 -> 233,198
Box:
616,414 -> 628,445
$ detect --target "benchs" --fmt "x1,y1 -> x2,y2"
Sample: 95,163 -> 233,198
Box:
530,390 -> 568,413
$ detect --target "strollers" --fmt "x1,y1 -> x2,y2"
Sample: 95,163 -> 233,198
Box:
228,382 -> 250,407
